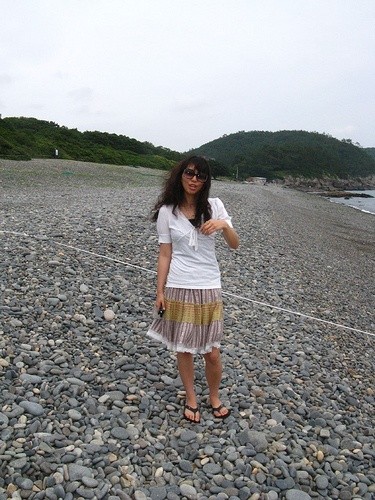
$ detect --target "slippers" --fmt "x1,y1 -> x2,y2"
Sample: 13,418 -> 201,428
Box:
211,401 -> 230,419
183,399 -> 200,423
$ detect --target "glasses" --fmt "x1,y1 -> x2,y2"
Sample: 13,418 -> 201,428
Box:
183,168 -> 210,182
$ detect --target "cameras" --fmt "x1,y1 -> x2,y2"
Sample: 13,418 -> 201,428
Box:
158,310 -> 164,317
